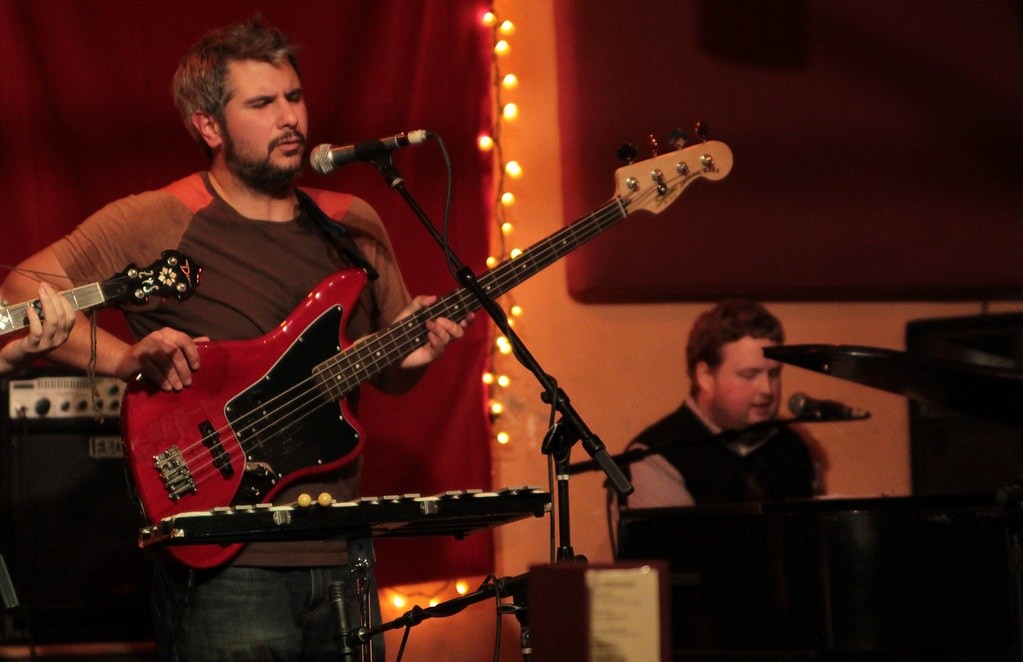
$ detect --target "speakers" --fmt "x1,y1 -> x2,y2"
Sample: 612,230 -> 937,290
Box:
0,363 -> 167,646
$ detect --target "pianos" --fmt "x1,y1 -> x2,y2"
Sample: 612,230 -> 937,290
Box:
612,311 -> 1023,662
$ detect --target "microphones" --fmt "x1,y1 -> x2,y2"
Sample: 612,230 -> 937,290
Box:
325,581 -> 355,662
787,392 -> 870,420
309,131 -> 436,173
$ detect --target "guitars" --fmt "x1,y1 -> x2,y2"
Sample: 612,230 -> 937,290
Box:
118,118 -> 736,572
0,247 -> 203,339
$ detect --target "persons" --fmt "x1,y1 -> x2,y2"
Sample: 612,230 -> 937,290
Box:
0,14 -> 475,662
620,296 -> 816,509
